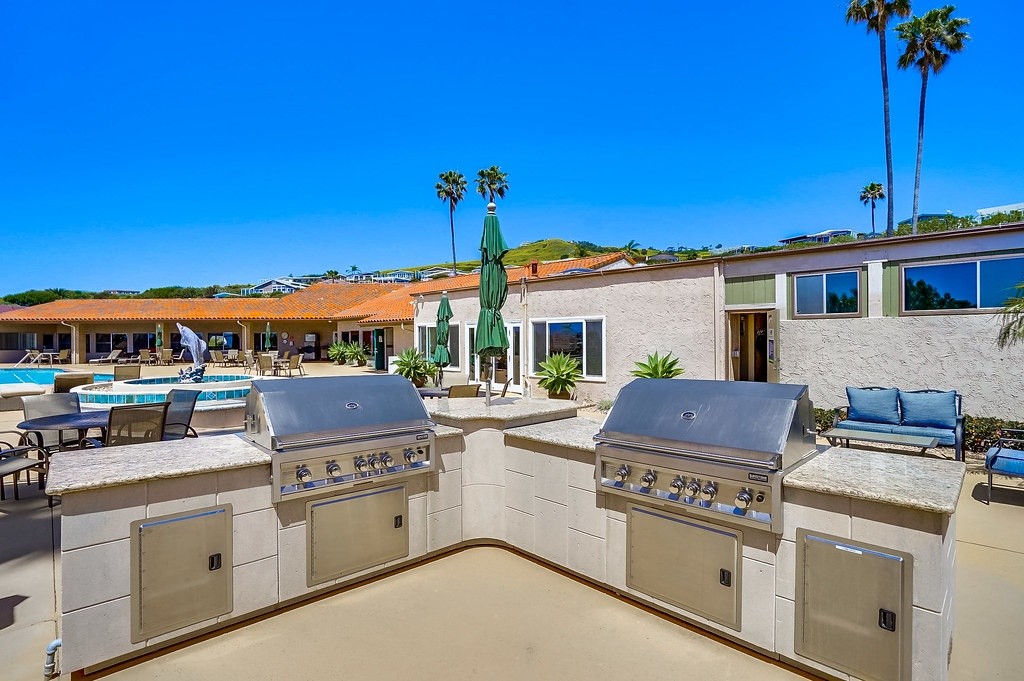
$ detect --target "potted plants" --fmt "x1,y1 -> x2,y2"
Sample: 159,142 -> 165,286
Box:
343,341 -> 369,366
327,340 -> 350,365
390,347 -> 440,387
534,349 -> 584,400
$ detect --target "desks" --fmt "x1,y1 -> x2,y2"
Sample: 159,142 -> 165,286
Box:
222,353 -> 239,367
14,411 -> 162,452
418,387 -> 498,400
272,359 -> 290,376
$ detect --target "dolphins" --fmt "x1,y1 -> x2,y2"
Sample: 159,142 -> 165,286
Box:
176,322 -> 207,375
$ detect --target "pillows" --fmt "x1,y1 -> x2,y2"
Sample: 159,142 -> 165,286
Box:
846,386 -> 901,424
897,389 -> 958,428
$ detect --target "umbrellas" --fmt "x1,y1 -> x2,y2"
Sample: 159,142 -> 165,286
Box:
433,290 -> 453,398
156,324 -> 163,365
265,322 -> 271,353
475,201 -> 510,406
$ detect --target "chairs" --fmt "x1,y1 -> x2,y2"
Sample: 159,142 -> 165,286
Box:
0,348 -> 202,504
984,428 -> 1024,505
501,377 -> 513,397
208,348 -> 306,378
448,383 -> 482,398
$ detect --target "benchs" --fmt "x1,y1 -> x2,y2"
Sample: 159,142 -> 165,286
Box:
821,427 -> 941,456
832,387 -> 966,462
